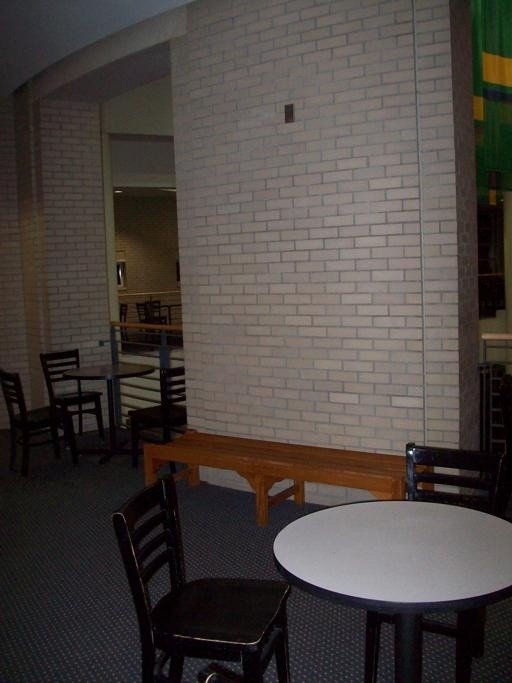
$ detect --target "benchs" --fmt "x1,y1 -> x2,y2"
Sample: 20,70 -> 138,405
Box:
143,429 -> 435,528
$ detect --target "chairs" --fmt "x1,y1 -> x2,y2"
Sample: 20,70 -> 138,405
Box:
119,299 -> 169,348
127,364 -> 187,474
40,349 -> 105,450
111,473 -> 292,683
364,442 -> 512,683
0,369 -> 78,479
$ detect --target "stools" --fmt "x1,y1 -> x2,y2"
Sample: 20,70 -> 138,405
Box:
481,332 -> 512,363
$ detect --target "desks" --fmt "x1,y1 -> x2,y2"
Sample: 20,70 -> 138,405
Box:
152,304 -> 182,339
272,499 -> 512,683
62,362 -> 154,466
